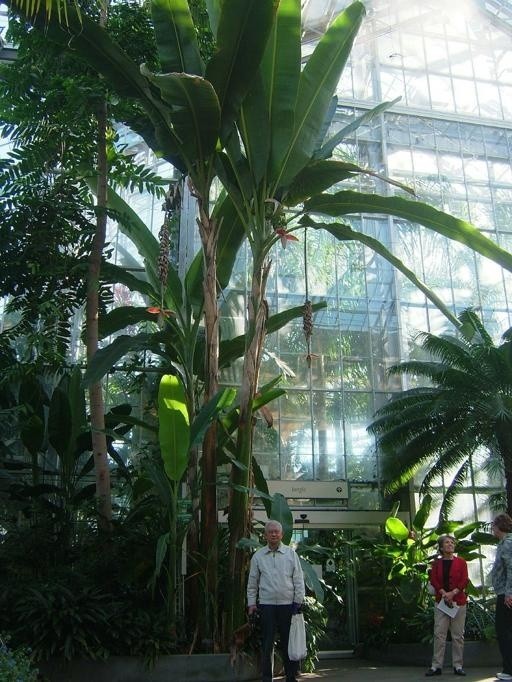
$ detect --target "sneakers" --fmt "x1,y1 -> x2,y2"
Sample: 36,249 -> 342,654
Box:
495,672 -> 512,680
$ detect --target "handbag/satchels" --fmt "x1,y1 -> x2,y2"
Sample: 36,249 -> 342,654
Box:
287,610 -> 308,662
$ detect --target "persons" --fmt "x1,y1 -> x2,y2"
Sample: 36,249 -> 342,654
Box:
247,520 -> 307,681
488,514 -> 511,680
423,536 -> 470,677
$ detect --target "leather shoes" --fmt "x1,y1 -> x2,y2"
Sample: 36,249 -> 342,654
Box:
454,667 -> 467,677
424,668 -> 443,677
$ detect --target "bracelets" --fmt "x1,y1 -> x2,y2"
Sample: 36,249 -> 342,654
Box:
453,588 -> 458,594
